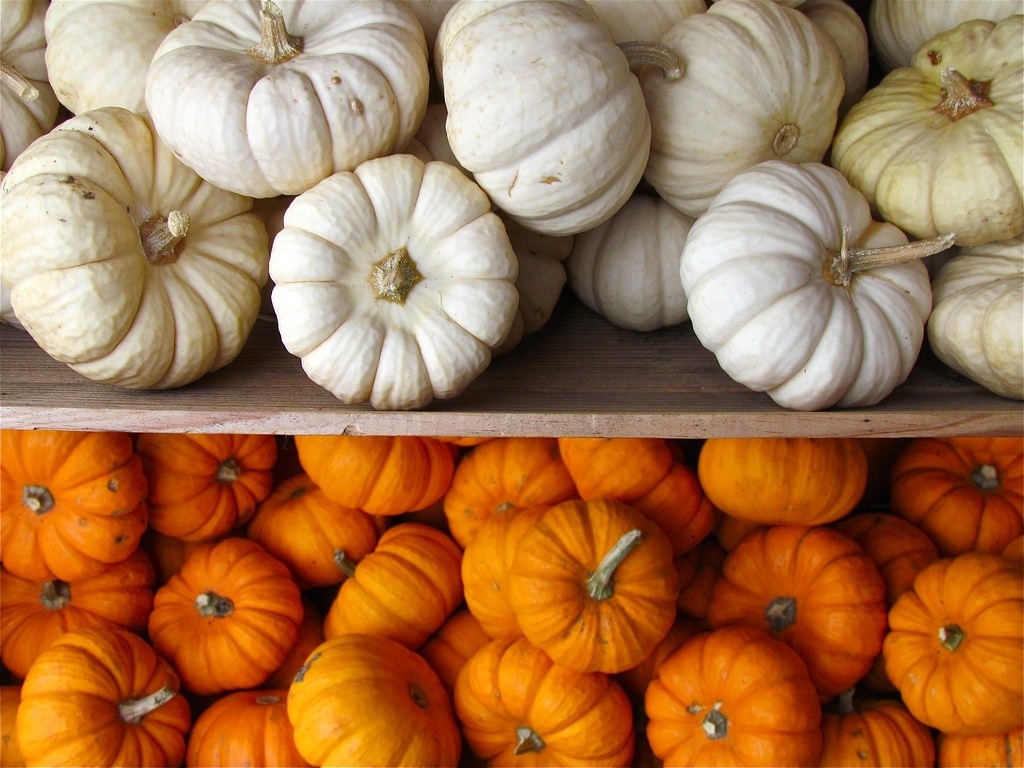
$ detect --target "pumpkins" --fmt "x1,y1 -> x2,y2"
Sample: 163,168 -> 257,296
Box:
1,0 -> 1024,768
